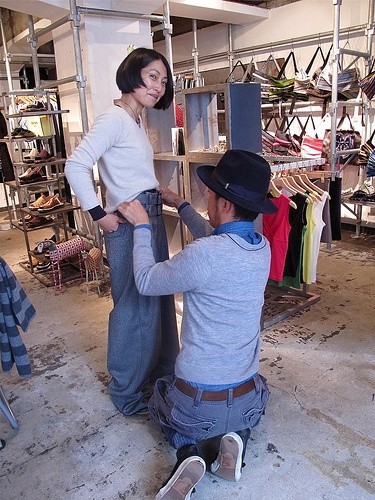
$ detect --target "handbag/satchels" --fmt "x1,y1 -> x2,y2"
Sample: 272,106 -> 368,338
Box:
220,41 -> 375,177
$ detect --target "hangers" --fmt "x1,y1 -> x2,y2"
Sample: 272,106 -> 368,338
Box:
267,159 -> 342,209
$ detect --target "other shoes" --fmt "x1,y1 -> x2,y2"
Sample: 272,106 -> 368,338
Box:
28,192 -> 64,212
19,102 -> 48,112
33,235 -> 66,258
27,258 -> 51,270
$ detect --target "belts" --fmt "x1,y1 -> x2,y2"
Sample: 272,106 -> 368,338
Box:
174,377 -> 256,402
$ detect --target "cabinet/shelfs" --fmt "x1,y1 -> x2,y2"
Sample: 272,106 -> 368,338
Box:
113,83 -> 263,316
173,21 -> 375,240
0,90 -> 90,287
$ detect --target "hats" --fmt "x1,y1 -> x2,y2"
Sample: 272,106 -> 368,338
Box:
196,149 -> 278,214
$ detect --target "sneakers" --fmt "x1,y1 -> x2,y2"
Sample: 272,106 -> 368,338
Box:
154,444 -> 206,500
210,428 -> 251,482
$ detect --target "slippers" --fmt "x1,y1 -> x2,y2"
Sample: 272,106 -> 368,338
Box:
18,167 -> 48,184
4,128 -> 36,139
17,214 -> 54,228
23,148 -> 56,164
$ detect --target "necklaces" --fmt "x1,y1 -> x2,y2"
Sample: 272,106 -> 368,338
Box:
121,100 -> 140,124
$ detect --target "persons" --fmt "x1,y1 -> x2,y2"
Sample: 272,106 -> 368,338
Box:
64,48 -> 179,415
118,149 -> 278,500
